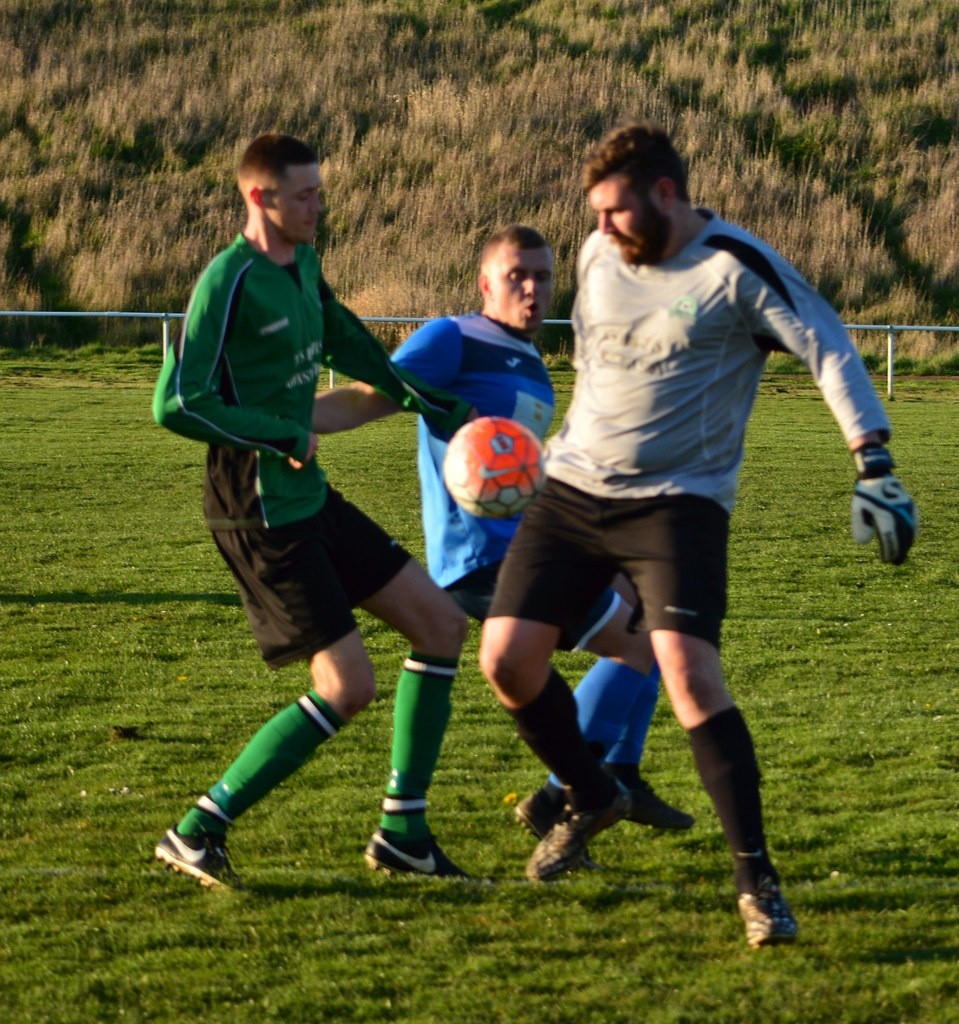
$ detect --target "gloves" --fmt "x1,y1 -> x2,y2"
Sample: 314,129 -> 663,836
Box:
849,442 -> 916,565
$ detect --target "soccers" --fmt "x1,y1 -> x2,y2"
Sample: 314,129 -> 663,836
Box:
444,416 -> 546,519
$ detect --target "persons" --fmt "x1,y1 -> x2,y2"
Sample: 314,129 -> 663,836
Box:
477,125 -> 917,945
309,224 -> 695,868
154,135 -> 487,888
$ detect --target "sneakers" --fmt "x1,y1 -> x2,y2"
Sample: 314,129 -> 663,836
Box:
513,788 -> 599,868
607,768 -> 695,830
153,824 -> 245,893
735,869 -> 797,948
363,830 -> 470,879
523,780 -> 632,882
